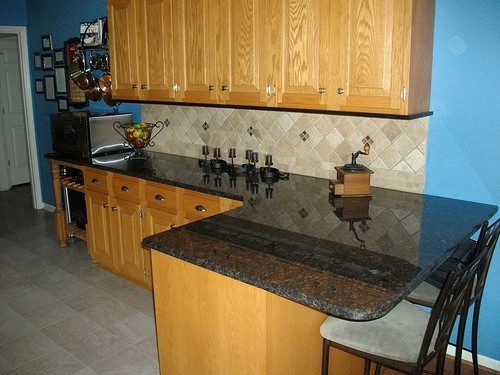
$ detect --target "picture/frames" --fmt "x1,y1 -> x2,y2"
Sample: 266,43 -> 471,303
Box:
53,65 -> 68,94
56,96 -> 70,113
34,78 -> 45,95
40,35 -> 52,51
33,54 -> 43,71
43,75 -> 57,101
41,53 -> 54,71
52,48 -> 65,65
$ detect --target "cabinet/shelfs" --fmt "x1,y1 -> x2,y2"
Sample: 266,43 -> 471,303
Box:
83,166 -> 145,289
43,152 -> 96,265
107,0 -> 434,120
144,182 -> 244,292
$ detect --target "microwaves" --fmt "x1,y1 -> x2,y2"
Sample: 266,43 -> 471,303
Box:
49,110 -> 133,160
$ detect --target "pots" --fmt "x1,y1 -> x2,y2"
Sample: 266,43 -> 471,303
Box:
68,50 -> 121,110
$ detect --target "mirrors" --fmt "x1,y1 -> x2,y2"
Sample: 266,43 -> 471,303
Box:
62,37 -> 89,108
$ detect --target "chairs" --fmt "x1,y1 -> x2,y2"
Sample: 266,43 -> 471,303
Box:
320,248 -> 490,375
374,216 -> 500,375
